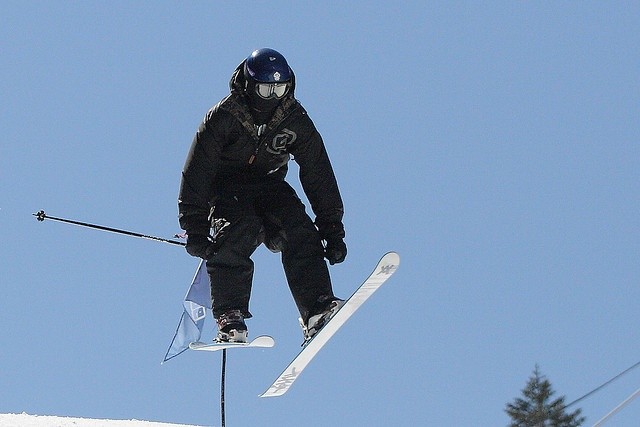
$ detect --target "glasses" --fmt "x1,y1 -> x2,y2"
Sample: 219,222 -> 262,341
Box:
254,82 -> 290,100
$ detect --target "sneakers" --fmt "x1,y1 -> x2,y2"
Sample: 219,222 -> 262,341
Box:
216,308 -> 248,342
302,297 -> 344,341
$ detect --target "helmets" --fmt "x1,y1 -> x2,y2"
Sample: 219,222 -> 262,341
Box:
244,48 -> 292,97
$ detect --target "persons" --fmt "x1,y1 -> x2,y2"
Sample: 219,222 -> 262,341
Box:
177,48 -> 347,345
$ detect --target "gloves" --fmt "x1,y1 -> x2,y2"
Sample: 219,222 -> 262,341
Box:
186,223 -> 215,260
318,222 -> 347,265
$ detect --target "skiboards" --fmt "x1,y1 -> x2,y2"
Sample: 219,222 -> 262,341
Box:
188,252 -> 400,397
